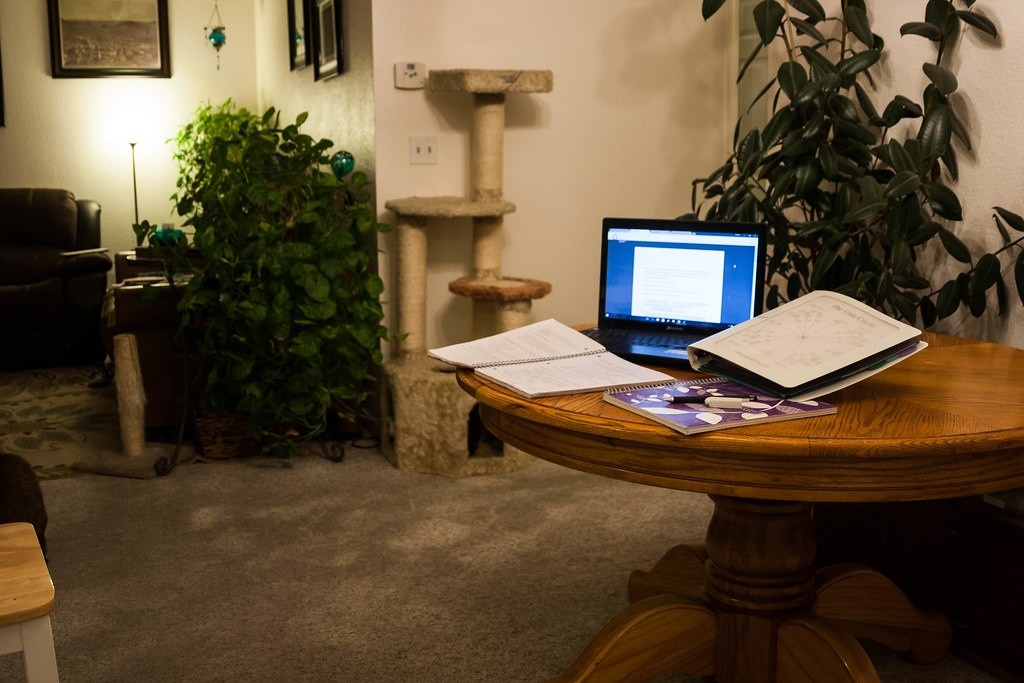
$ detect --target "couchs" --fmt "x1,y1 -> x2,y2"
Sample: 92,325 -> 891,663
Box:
0,188 -> 113,376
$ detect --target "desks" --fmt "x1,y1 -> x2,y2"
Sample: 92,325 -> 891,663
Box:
457,322 -> 1023,682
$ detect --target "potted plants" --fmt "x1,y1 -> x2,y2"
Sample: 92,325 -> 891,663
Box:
129,96 -> 409,476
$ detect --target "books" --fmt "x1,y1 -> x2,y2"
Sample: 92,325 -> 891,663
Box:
426,318 -> 678,400
603,377 -> 838,437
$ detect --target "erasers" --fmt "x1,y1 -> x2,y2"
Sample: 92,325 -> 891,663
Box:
704,396 -> 743,409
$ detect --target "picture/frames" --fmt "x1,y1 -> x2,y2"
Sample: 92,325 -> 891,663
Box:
47,0 -> 170,78
313,0 -> 343,82
286,0 -> 311,71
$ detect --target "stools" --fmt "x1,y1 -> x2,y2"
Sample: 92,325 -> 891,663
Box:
0,523 -> 60,683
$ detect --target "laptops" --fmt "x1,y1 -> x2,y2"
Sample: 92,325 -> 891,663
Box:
577,217 -> 767,365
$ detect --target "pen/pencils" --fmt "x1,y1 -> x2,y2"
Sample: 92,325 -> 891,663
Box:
663,395 -> 760,404
